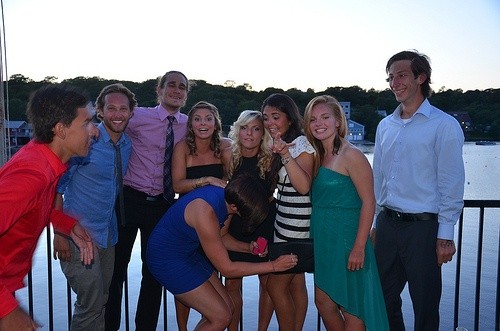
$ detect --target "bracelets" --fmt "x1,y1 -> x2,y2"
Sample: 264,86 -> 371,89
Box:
270,261 -> 275,272
192,176 -> 207,188
282,156 -> 293,164
281,151 -> 289,156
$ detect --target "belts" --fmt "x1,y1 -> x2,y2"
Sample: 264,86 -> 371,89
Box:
381,206 -> 436,221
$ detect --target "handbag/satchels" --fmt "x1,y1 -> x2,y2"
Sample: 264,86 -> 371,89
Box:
269,239 -> 315,274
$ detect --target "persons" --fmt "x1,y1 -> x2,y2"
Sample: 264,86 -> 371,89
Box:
88,71 -> 194,331
146,174 -> 298,331
0,82 -> 95,331
171,101 -> 231,330
262,93 -> 316,331
305,94 -> 389,331
53,84 -> 137,331
223,110 -> 274,331
371,51 -> 465,331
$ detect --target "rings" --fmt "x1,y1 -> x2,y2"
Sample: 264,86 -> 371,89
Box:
292,258 -> 295,263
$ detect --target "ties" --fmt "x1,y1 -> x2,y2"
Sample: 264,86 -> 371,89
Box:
110,143 -> 126,230
163,115 -> 174,204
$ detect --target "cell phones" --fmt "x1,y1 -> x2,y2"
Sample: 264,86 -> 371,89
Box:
252,236 -> 268,255
